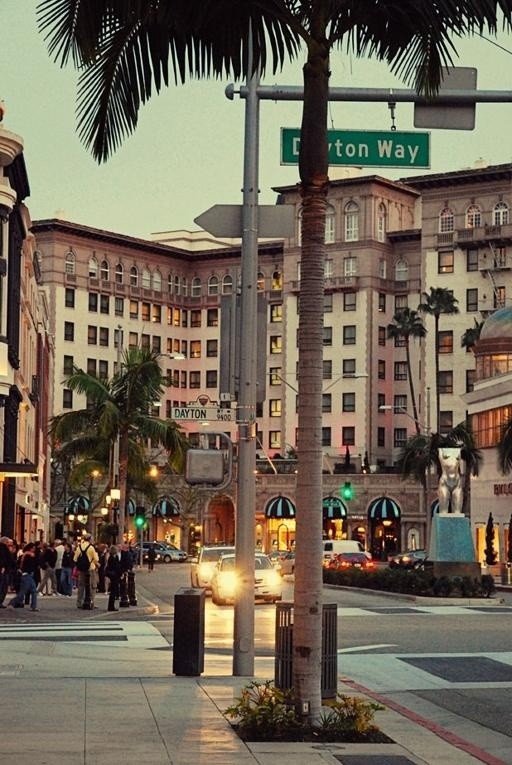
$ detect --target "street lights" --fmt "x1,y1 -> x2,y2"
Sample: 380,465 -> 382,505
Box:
112,350 -> 187,546
267,368 -> 369,398
376,390 -> 431,559
67,487 -> 121,545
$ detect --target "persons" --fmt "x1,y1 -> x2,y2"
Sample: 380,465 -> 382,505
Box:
147,544 -> 156,574
1,535 -> 135,612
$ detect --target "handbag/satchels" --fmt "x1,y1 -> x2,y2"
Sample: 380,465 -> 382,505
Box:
104,567 -> 116,577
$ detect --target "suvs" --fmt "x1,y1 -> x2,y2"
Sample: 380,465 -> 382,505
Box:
133,541 -> 189,563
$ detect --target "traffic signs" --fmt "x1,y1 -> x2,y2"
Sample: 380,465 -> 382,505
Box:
280,126 -> 428,168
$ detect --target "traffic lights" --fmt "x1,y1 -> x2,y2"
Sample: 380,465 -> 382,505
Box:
135,506 -> 146,527
342,481 -> 352,502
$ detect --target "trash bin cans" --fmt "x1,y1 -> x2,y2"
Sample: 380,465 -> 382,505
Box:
275,601 -> 337,702
173,587 -> 206,676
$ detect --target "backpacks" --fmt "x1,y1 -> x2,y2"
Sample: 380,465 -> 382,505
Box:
77,544 -> 92,571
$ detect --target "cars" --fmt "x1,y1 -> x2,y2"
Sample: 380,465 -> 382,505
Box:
190,545 -> 236,592
210,551 -> 284,607
268,537 -> 426,576
